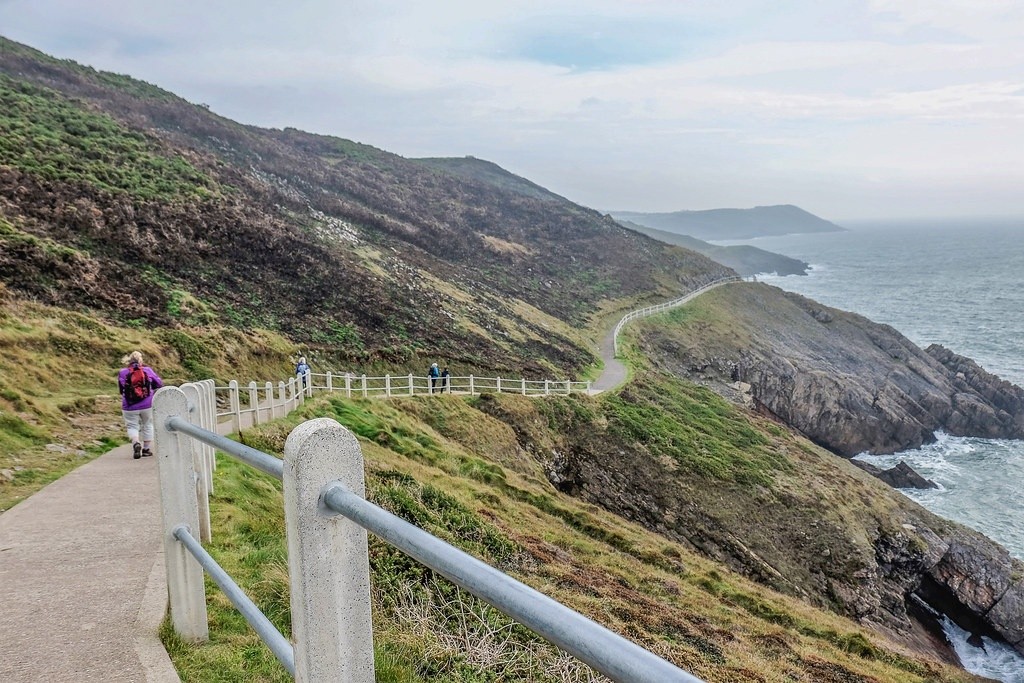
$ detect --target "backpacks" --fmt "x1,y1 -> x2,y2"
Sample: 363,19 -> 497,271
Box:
442,372 -> 447,380
298,365 -> 307,379
122,364 -> 151,407
429,368 -> 434,377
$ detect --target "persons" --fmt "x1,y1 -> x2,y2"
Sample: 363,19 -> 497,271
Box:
290,350 -> 306,388
118,351 -> 162,460
428,363 -> 452,394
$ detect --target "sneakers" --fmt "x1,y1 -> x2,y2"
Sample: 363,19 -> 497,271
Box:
133,442 -> 142,459
141,448 -> 154,456
